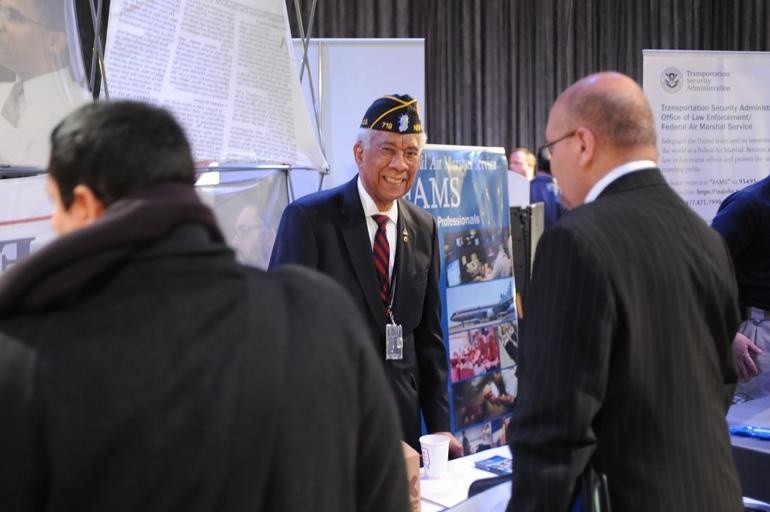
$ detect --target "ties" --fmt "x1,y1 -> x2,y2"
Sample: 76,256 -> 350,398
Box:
371,214 -> 392,322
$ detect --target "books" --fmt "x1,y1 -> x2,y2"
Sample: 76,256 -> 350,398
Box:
473,455 -> 514,476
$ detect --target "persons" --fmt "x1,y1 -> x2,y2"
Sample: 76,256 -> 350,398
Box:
0,101 -> 410,509
508,145 -> 537,184
0,0 -> 99,172
710,172 -> 769,434
448,321 -> 519,455
529,145 -> 567,232
507,71 -> 747,510
267,93 -> 466,457
445,229 -> 509,285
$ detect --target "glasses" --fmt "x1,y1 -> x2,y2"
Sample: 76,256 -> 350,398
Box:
538,131 -> 575,161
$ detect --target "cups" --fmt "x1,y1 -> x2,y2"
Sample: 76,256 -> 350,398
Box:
419,433 -> 451,480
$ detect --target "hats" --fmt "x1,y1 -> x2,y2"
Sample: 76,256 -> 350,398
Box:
360,94 -> 424,135
